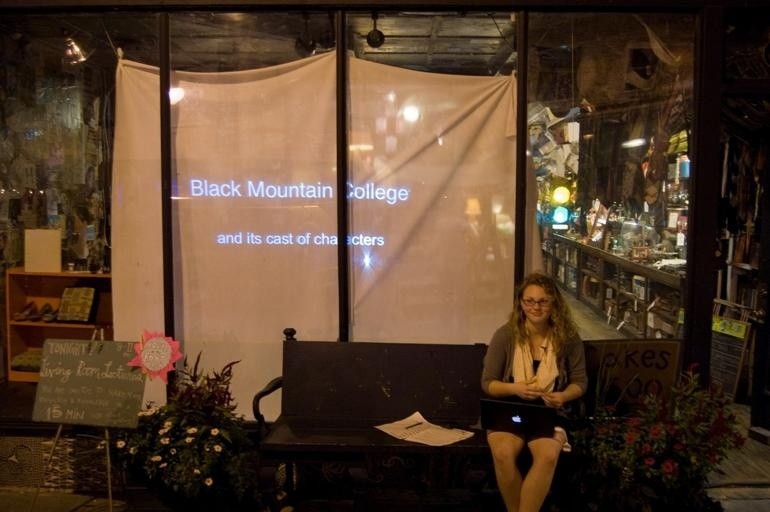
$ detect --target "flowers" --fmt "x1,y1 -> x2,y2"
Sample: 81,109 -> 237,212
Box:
590,361 -> 746,491
109,353 -> 264,506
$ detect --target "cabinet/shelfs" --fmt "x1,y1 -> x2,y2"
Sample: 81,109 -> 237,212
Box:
539,232 -> 687,339
5,267 -> 112,383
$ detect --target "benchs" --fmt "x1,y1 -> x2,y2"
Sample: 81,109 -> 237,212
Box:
252,328 -> 501,512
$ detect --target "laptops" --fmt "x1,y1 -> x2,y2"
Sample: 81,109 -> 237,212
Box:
479,398 -> 555,438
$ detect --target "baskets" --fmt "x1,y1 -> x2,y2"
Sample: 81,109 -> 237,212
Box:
41,439 -> 124,493
0,436 -> 49,485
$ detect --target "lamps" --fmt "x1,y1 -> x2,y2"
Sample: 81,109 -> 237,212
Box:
294,11 -> 385,58
55,25 -> 95,65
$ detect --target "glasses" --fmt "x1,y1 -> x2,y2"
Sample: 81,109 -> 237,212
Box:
520,298 -> 551,307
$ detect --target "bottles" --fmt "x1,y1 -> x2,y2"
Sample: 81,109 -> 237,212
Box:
680,155 -> 691,177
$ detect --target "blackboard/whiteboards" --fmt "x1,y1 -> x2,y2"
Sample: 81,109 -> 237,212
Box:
32,339 -> 146,428
670,308 -> 753,406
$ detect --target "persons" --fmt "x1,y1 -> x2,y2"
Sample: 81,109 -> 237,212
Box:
481,273 -> 588,511
530,118 -> 553,174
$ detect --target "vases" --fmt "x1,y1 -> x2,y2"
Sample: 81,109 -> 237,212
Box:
636,484 -> 704,512
164,490 -> 226,511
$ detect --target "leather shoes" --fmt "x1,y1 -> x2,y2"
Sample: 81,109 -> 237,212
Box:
87,246 -> 111,272
14,301 -> 58,321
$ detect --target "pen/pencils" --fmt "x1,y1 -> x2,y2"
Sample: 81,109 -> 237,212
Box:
406,422 -> 423,429
